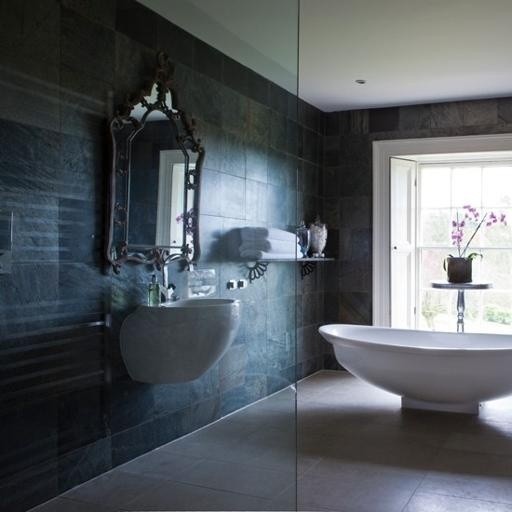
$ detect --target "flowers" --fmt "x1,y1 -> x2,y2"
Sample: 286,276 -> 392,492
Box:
442,203 -> 508,271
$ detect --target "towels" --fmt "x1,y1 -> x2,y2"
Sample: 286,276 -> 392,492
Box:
238,226 -> 303,260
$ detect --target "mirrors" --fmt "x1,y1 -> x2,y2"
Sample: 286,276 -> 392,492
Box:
103,51 -> 206,275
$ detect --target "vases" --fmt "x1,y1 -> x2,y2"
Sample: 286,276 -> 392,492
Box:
447,257 -> 473,283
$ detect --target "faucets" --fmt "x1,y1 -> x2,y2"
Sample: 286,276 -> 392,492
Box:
159,252 -> 194,303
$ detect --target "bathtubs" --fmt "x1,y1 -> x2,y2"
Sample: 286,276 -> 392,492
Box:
318,322 -> 512,415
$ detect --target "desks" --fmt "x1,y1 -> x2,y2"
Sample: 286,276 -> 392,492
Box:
430,282 -> 494,334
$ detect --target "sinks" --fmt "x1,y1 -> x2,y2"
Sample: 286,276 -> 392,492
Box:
119,299 -> 239,384
